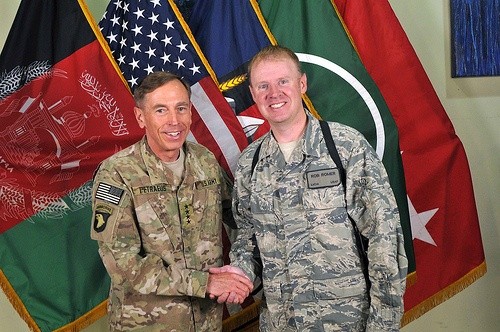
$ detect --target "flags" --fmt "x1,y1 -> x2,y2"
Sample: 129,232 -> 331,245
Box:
0,0 -> 488,332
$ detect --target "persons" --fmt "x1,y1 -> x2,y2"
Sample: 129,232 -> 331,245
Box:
208,44 -> 410,332
89,71 -> 254,332
